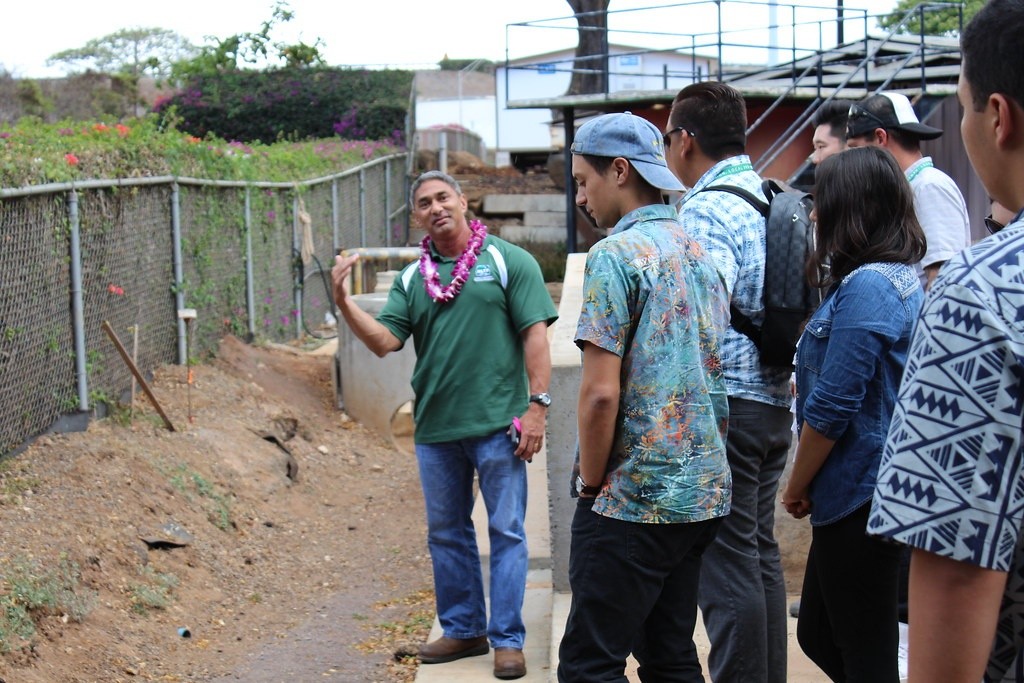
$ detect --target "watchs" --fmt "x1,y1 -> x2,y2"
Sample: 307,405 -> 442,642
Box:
529,393 -> 551,407
575,476 -> 599,495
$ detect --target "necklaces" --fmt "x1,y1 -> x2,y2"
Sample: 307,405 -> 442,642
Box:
420,221 -> 488,302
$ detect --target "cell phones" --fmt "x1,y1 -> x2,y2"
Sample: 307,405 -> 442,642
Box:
511,424 -> 532,463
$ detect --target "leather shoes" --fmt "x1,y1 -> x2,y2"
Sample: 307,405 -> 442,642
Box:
493,647 -> 526,678
416,634 -> 489,661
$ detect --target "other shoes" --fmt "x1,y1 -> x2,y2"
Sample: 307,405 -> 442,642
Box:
789,601 -> 800,617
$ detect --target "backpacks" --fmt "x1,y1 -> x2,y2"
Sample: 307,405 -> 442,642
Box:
701,180 -> 815,372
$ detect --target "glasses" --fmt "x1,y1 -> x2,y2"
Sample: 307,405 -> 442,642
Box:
848,104 -> 887,130
984,214 -> 1005,235
663,127 -> 694,147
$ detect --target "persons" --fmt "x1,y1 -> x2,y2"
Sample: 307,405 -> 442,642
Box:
865,0 -> 1024,682
558,110 -> 734,683
330,171 -> 560,679
983,198 -> 1018,236
847,93 -> 971,290
810,99 -> 852,165
663,81 -> 793,683
783,146 -> 928,683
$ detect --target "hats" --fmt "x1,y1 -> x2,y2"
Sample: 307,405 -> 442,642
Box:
570,111 -> 686,191
845,93 -> 944,141
